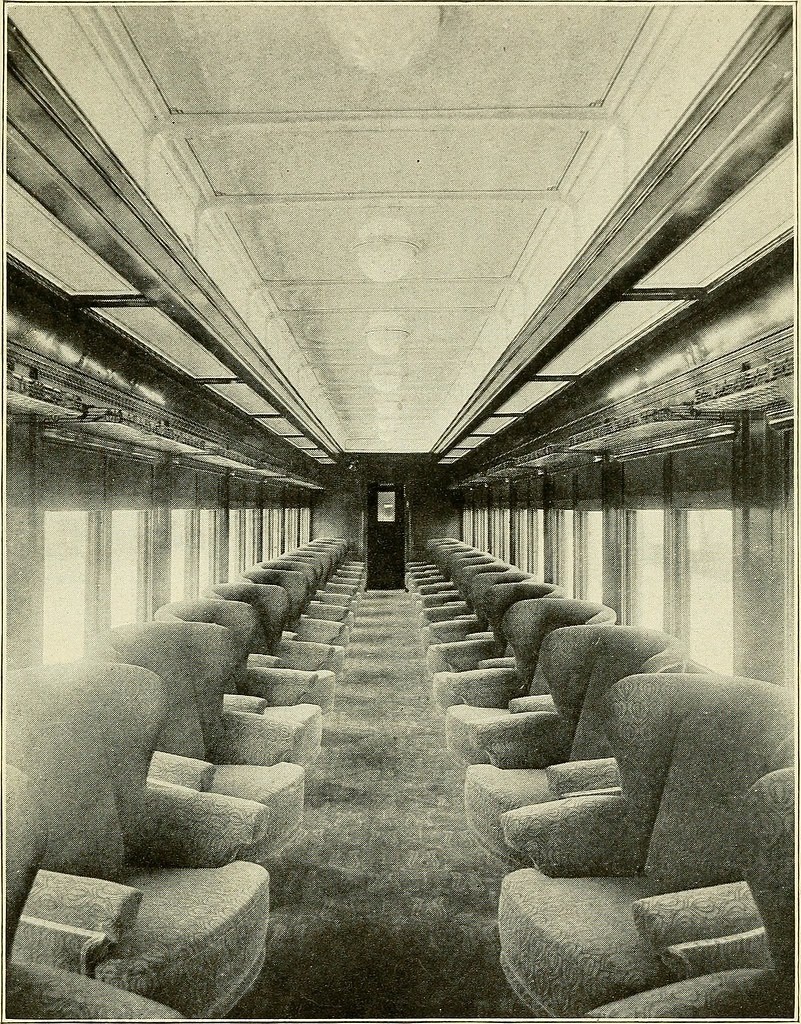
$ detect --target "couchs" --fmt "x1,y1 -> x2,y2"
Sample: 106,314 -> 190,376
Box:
499,671 -> 794,1019
447,599 -> 616,766
6,663 -> 271,1019
153,599 -> 322,771
87,620 -> 306,863
582,767 -> 794,1019
6,763 -> 184,1019
428,572 -> 539,678
434,582 -> 566,708
416,557 -> 504,616
205,583 -> 335,724
235,570 -> 346,679
405,538 -> 491,607
253,537 -> 366,644
419,564 -> 519,629
463,621 -> 687,869
